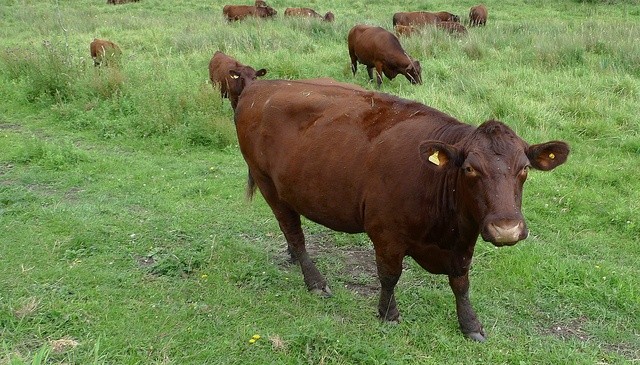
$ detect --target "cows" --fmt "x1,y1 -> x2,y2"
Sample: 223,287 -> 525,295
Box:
89,37 -> 124,70
222,0 -> 279,24
233,75 -> 570,345
392,11 -> 469,40
282,7 -> 335,26
467,5 -> 488,27
206,50 -> 269,112
346,22 -> 425,88
